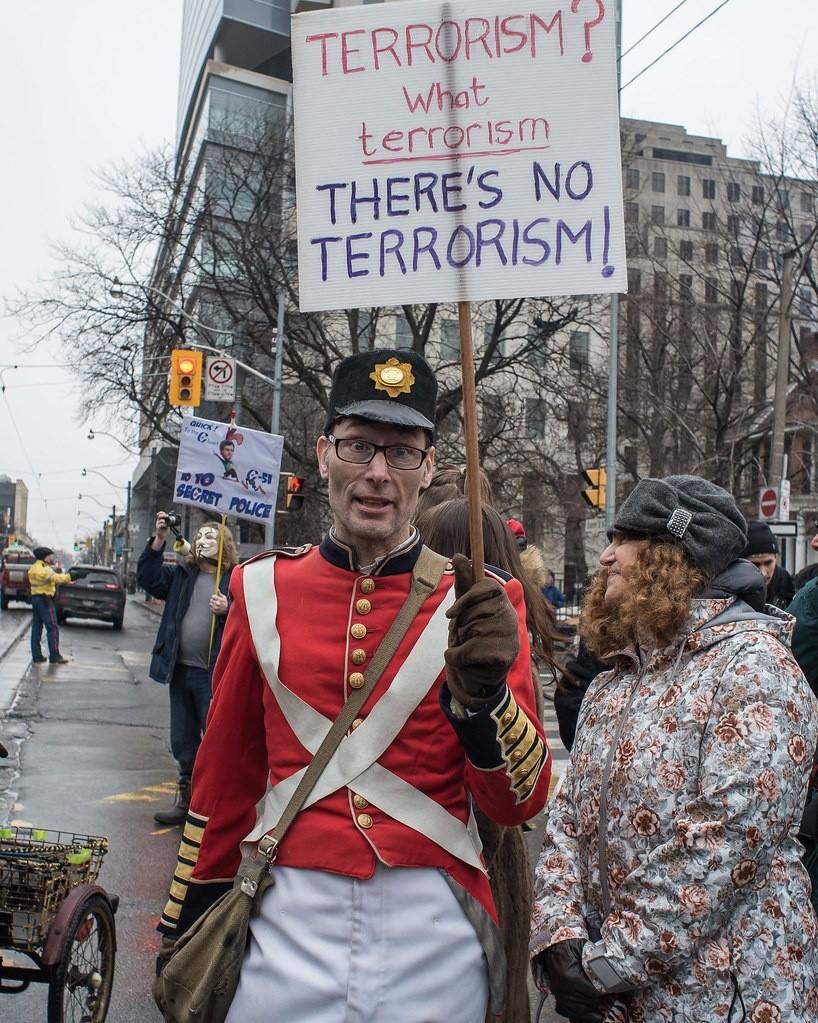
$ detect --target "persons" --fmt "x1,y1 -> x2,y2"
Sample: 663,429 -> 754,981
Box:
554,639 -> 614,751
220,440 -> 235,461
28,548 -> 74,665
157,351 -> 554,1022
541,570 -> 563,607
136,511 -> 240,823
784,534 -> 818,700
409,467 -> 575,1023
528,475 -> 818,1023
741,521 -> 794,611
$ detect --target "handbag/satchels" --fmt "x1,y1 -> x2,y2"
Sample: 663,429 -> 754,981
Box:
151,872 -> 260,1023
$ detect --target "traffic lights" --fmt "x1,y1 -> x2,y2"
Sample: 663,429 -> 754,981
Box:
74,542 -> 78,550
580,468 -> 606,510
169,350 -> 202,406
287,476 -> 305,511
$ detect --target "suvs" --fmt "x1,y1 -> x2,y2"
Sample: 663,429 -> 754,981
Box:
53,564 -> 126,628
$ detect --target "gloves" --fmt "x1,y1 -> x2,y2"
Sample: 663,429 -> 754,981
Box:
155,935 -> 182,975
445,554 -> 517,709
533,937 -> 617,1023
78,569 -> 90,579
53,567 -> 61,574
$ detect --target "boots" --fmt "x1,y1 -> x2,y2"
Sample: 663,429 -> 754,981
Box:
152,781 -> 192,826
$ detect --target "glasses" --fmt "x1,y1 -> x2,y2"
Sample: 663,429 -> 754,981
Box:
328,435 -> 428,471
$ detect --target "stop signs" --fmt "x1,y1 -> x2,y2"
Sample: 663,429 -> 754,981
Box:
758,487 -> 779,519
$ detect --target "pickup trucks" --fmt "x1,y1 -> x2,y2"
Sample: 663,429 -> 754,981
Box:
0,554 -> 39,610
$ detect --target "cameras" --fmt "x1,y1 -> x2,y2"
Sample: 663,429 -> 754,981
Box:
164,510 -> 181,527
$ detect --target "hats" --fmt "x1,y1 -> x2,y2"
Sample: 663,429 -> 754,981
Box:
608,473 -> 749,581
33,547 -> 53,560
738,521 -> 780,557
506,519 -> 525,538
324,349 -> 438,434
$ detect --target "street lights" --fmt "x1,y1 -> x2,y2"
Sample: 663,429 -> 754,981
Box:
88,428 -> 156,601
110,275 -> 240,555
77,511 -> 106,565
79,493 -> 115,565
81,468 -> 131,586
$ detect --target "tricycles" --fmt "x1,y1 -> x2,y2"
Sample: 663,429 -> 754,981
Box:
0,743 -> 119,1023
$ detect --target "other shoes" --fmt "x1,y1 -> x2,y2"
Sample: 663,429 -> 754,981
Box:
32,655 -> 48,663
48,653 -> 68,663
